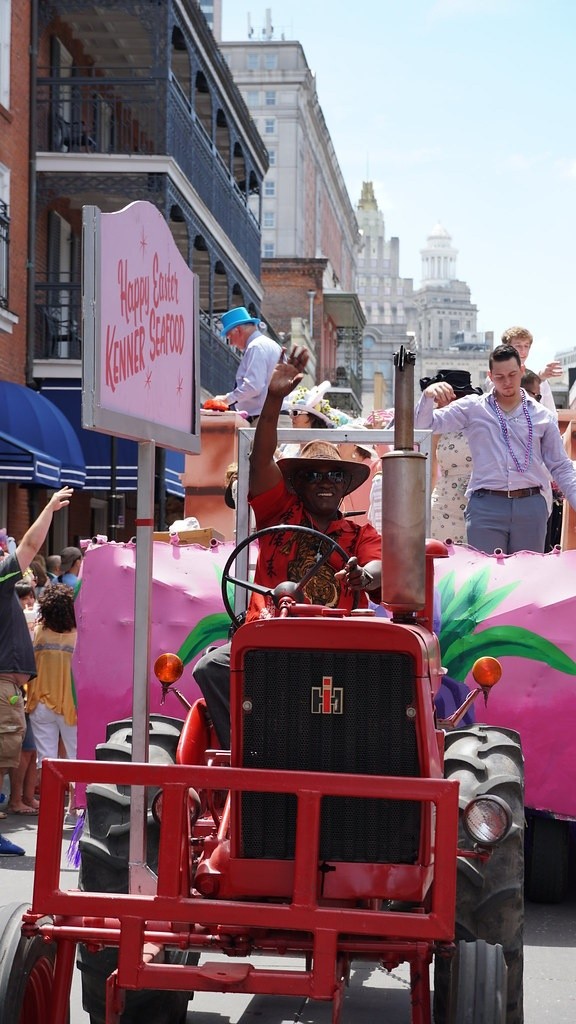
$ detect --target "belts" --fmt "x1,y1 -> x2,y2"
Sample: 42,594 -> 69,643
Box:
477,487 -> 541,498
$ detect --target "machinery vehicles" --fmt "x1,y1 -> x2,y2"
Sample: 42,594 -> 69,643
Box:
1,328 -> 537,1024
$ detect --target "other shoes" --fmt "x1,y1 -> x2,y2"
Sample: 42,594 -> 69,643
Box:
64,813 -> 79,824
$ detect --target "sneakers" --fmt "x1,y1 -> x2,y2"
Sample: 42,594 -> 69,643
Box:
0,834 -> 25,858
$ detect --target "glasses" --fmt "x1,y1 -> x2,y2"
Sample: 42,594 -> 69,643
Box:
290,410 -> 308,417
289,470 -> 345,484
526,389 -> 542,401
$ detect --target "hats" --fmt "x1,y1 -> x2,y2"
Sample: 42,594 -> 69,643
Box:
59,547 -> 82,572
218,307 -> 262,336
222,463 -> 238,510
281,380 -> 338,430
419,370 -> 483,397
277,439 -> 371,498
339,421 -> 377,456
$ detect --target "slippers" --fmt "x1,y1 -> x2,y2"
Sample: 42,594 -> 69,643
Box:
6,799 -> 39,816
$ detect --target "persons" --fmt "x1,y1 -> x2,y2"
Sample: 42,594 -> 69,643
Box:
0,307 -> 576,855
